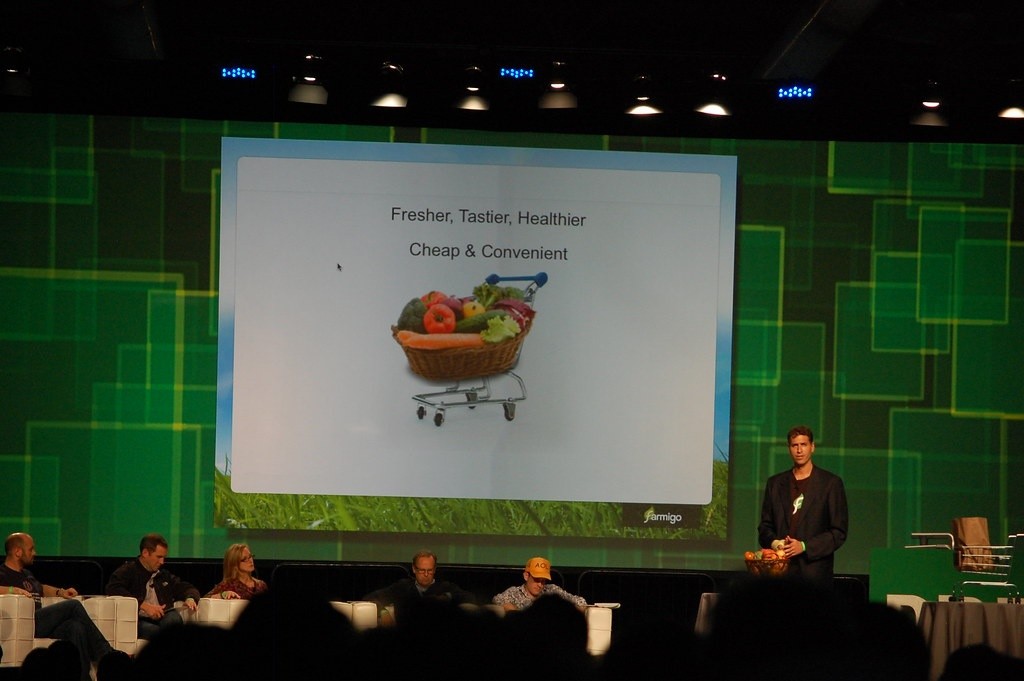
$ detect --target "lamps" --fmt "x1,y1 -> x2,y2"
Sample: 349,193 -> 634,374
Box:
278,53 -> 1024,126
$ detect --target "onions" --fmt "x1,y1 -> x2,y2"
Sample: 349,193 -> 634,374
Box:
440,294 -> 464,320
496,298 -> 536,331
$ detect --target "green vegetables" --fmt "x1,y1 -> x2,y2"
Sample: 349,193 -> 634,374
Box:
470,282 -> 525,343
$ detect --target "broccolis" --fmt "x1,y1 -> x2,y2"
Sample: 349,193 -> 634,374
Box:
398,297 -> 428,335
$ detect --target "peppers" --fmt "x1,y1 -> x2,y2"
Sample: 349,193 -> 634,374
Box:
420,289 -> 482,334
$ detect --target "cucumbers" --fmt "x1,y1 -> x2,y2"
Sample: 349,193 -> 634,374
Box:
455,309 -> 507,332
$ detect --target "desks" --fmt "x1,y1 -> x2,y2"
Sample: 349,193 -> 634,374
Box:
917,602 -> 1024,681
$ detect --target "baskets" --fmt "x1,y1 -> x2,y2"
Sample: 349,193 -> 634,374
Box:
745,553 -> 790,577
394,299 -> 532,381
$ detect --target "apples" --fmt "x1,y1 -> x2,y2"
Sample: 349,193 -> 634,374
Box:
745,548 -> 789,575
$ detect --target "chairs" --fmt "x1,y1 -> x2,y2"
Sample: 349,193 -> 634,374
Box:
887,593 -> 929,626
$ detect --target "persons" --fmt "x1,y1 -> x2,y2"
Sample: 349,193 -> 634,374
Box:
758,426 -> 848,576
0,532 -> 113,681
106,533 -> 200,642
366,549 -> 481,626
202,543 -> 267,599
493,557 -> 588,614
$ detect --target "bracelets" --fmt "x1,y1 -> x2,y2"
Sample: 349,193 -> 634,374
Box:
801,541 -> 806,552
380,610 -> 390,615
56,589 -> 60,597
9,586 -> 14,594
186,598 -> 194,601
221,591 -> 227,599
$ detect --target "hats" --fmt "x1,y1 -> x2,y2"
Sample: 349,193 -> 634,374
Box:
526,555 -> 553,581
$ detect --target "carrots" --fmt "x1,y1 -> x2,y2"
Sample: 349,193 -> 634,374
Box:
398,330 -> 483,349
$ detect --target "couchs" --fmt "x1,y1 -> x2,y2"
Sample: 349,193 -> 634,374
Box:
0,594 -> 612,681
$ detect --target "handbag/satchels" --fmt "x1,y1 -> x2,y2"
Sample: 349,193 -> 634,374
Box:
954,516 -> 993,570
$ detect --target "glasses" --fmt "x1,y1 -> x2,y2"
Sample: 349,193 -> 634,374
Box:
414,564 -> 435,574
240,553 -> 255,563
